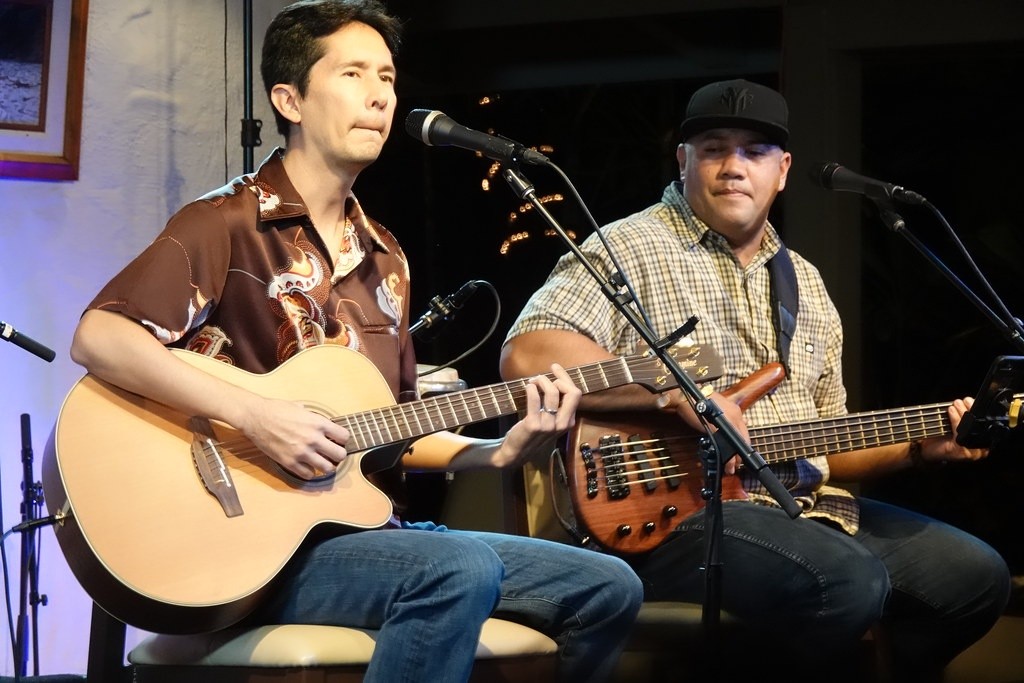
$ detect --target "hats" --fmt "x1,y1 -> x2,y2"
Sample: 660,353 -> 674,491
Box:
680,78 -> 790,149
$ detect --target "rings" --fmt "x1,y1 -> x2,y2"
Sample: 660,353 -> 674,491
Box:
543,406 -> 558,415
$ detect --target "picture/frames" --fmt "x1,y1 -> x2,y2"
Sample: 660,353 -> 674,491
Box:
0,0 -> 94,187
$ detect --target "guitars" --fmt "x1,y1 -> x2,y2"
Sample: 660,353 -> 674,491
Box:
41,342 -> 1024,637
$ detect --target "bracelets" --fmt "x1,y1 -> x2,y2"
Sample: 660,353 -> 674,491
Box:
909,439 -> 947,470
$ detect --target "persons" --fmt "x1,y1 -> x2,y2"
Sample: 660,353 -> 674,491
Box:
69,1 -> 645,682
499,79 -> 1011,682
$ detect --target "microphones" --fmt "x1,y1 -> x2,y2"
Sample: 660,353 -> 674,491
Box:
809,159 -> 926,205
405,108 -> 549,167
